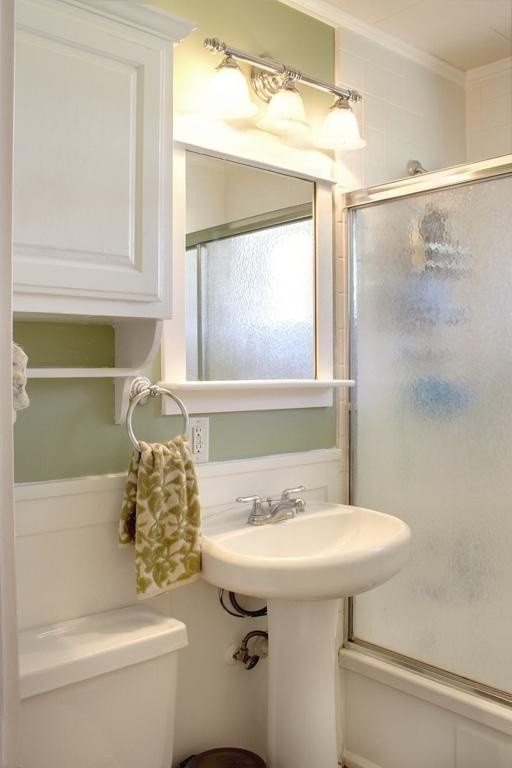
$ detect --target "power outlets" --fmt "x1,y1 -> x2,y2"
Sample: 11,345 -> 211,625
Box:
187,416 -> 209,464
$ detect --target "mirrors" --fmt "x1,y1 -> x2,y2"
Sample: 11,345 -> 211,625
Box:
184,149 -> 317,380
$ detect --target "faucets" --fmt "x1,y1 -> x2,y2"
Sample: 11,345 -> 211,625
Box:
267,497 -> 303,520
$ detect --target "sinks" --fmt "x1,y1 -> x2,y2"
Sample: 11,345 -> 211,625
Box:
201,508 -> 412,603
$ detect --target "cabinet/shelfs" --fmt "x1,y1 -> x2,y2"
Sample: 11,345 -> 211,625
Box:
13,1 -> 197,303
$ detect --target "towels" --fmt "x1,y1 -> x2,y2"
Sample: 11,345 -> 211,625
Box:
117,437 -> 203,600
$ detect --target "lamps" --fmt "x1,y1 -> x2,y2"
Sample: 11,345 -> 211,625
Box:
253,78 -> 312,135
185,54 -> 257,121
307,98 -> 368,152
